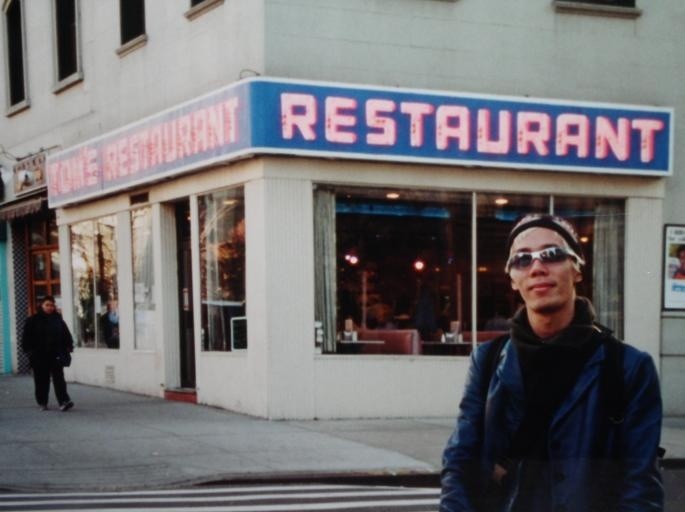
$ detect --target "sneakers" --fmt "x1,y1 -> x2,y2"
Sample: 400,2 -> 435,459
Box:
36,401 -> 73,412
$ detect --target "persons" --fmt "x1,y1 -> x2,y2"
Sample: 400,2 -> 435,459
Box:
100,301 -> 120,349
21,294 -> 75,411
438,210 -> 667,512
671,244 -> 685,279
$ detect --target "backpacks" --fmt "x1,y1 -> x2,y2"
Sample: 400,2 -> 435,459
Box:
481,333 -> 667,511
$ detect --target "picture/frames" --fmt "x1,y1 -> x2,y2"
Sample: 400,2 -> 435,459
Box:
663,222 -> 685,311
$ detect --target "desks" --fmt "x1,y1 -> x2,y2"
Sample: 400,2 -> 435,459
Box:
336,340 -> 484,355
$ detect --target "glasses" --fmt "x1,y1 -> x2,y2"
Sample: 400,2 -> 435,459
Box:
504,247 -> 579,272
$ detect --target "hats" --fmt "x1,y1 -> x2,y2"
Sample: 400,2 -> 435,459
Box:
504,211 -> 581,257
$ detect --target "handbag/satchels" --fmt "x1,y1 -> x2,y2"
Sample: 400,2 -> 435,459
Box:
52,350 -> 71,366
